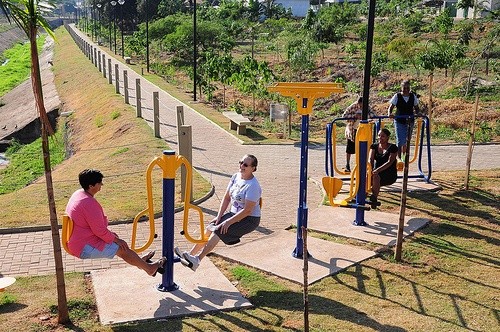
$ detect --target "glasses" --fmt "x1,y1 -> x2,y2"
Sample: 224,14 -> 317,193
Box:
239,161 -> 251,167
403,87 -> 409,90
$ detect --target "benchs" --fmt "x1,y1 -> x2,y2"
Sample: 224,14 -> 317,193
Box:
124,57 -> 132,64
223,110 -> 253,135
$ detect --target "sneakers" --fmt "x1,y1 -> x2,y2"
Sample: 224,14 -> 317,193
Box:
183,251 -> 201,272
174,247 -> 185,258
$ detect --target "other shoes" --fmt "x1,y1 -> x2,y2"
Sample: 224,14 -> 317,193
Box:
138,250 -> 155,269
370,194 -> 377,209
345,165 -> 350,172
149,257 -> 167,276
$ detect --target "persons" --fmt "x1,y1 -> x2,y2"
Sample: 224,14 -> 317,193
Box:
388,81 -> 422,161
342,97 -> 375,173
174,154 -> 261,272
355,129 -> 398,209
66,169 -> 167,277
